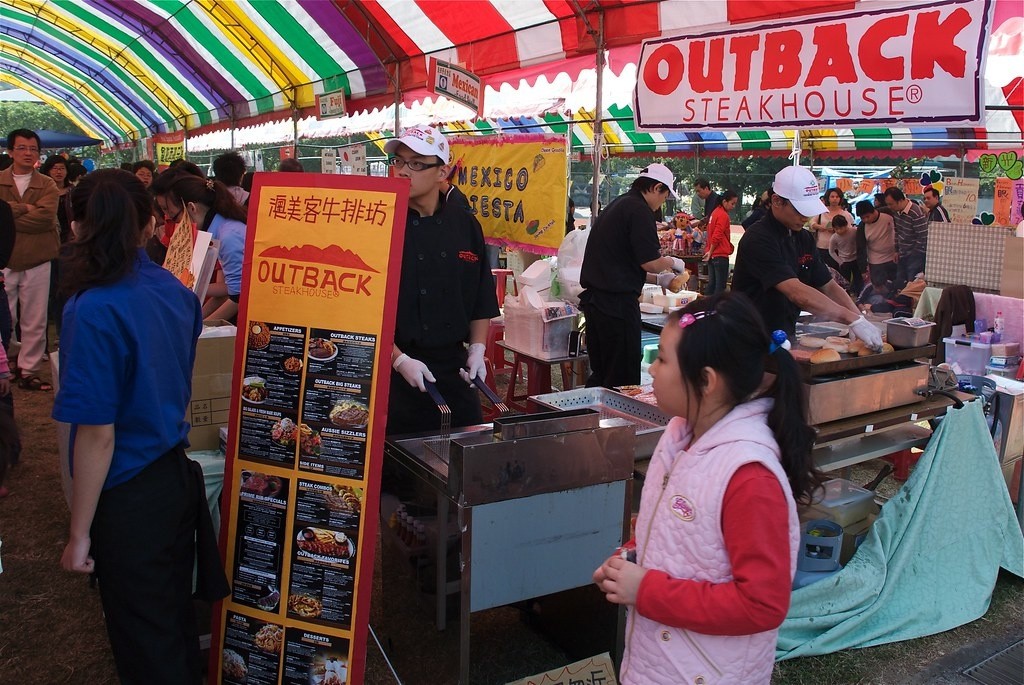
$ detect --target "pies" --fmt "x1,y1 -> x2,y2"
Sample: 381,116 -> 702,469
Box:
799,335 -> 851,353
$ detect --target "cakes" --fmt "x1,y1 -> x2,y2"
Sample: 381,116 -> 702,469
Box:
245,321 -> 270,349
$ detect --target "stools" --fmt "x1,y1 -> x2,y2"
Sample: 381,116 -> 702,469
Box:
476,267 -> 570,421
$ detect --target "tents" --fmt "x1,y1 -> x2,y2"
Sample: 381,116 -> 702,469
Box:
185,79 -> 1024,209
0,130 -> 103,159
0,0 -> 859,256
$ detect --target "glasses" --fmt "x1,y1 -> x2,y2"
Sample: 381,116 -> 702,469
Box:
388,157 -> 445,171
51,168 -> 66,171
13,145 -> 38,152
169,201 -> 197,223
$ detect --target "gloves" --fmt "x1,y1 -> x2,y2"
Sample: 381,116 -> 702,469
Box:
670,257 -> 685,274
393,352 -> 436,392
848,315 -> 883,352
458,343 -> 487,389
657,273 -> 684,293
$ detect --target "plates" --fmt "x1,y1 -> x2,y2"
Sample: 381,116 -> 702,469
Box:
297,527 -> 353,560
242,377 -> 267,404
308,339 -> 338,361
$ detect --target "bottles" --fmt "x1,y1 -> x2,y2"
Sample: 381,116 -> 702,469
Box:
994,312 -> 1004,340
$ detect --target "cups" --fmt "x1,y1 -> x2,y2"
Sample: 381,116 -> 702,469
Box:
980,332 -> 993,343
974,320 -> 984,334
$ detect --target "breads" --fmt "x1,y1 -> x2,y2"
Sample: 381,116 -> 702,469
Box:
669,271 -> 690,292
809,339 -> 894,362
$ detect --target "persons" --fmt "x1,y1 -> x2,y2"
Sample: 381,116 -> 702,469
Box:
702,190 -> 738,296
731,165 -> 883,352
589,200 -> 602,215
566,197 -> 576,234
691,176 -> 721,220
50,169 -> 230,685
383,126 -> 502,439
591,292 -> 826,685
1015,204 -> 1024,237
577,163 -> 685,390
809,187 -> 952,314
741,187 -> 774,231
0,129 -> 304,497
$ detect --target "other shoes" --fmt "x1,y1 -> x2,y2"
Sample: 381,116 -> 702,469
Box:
8,454 -> 24,479
41,353 -> 49,361
0,487 -> 8,497
53,334 -> 61,351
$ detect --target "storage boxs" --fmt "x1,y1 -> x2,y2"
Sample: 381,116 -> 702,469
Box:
931,327 -> 1023,511
793,480 -> 888,598
178,319 -> 237,452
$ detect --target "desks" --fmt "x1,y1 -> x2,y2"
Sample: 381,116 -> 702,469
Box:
48,349 -> 233,599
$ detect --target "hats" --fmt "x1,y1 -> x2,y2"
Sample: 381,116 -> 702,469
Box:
638,162 -> 680,200
384,125 -> 449,164
773,166 -> 830,218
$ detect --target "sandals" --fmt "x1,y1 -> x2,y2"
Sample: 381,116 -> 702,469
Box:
7,356 -> 19,383
18,374 -> 53,390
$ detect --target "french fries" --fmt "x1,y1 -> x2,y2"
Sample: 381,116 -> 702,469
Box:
313,529 -> 338,546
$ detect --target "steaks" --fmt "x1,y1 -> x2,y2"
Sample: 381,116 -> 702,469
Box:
332,405 -> 369,424
